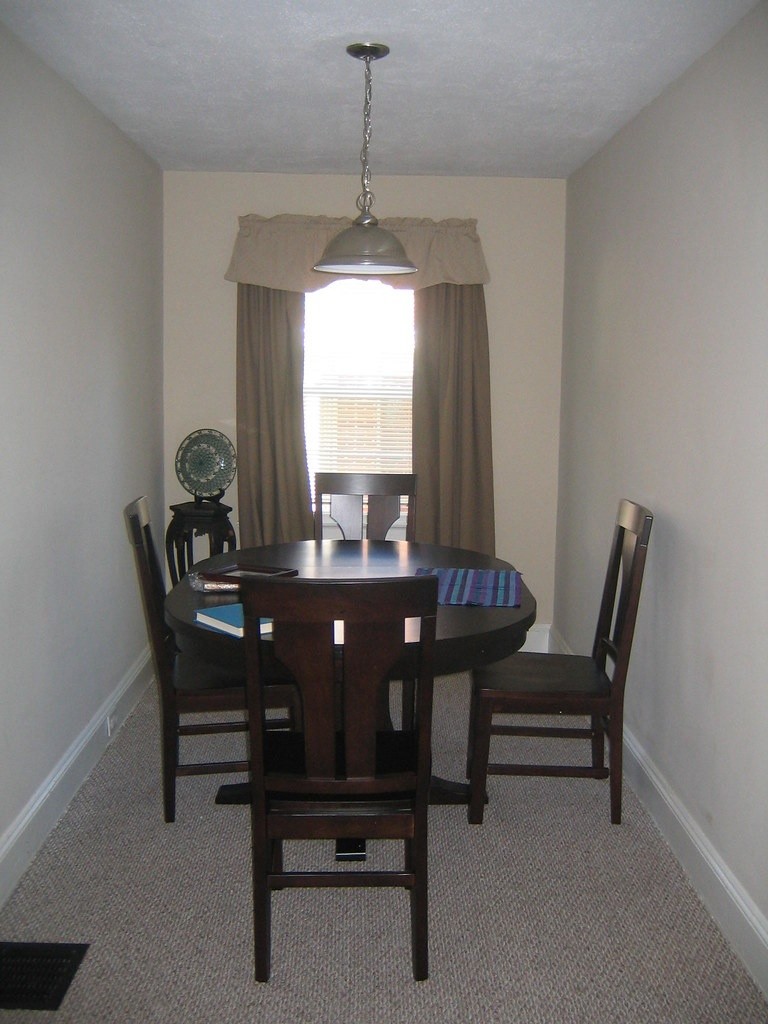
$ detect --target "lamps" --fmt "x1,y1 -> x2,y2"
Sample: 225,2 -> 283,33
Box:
314,43 -> 420,276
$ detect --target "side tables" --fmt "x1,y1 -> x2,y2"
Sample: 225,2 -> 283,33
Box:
164,501 -> 237,589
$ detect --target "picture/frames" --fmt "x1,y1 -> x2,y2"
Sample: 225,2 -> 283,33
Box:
206,564 -> 298,584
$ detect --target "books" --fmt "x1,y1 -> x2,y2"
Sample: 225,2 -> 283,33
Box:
193,603 -> 273,639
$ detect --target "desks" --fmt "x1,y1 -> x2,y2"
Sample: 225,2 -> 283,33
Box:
165,539 -> 537,804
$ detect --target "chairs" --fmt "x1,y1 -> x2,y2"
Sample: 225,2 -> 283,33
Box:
236,574 -> 439,984
466,498 -> 654,824
125,494 -> 302,824
313,471 -> 417,544
